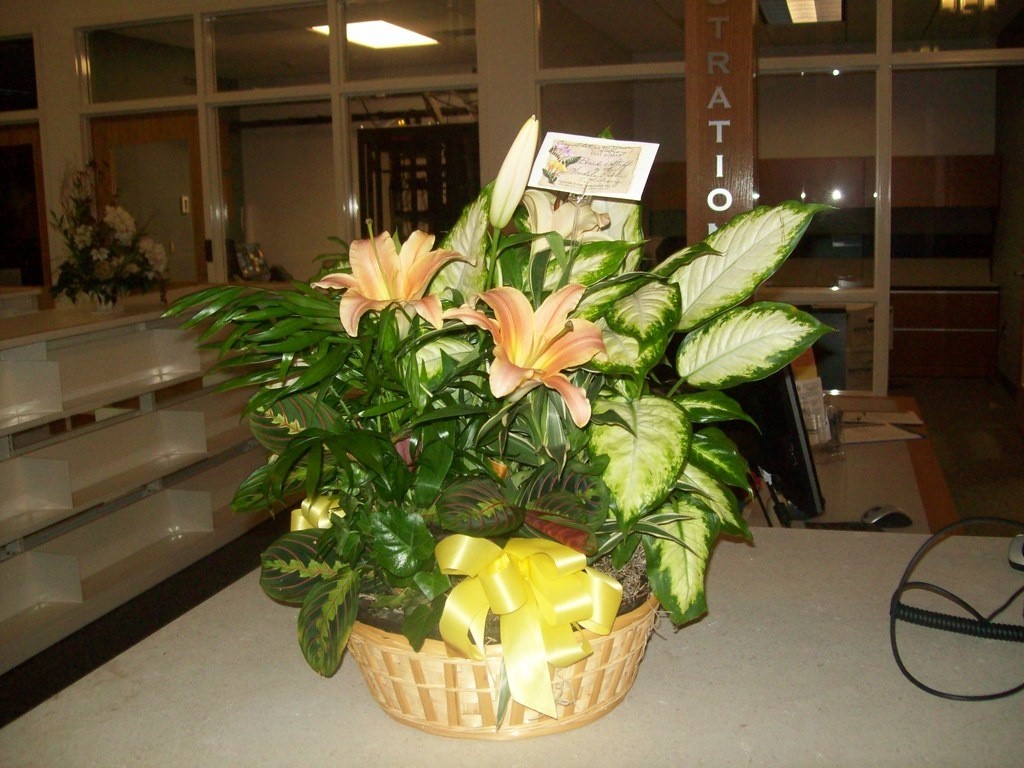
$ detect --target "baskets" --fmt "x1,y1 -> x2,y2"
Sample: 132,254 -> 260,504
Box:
346,592 -> 659,740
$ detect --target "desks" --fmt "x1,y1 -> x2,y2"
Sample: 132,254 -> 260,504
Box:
0,526 -> 1024,768
743,396 -> 964,535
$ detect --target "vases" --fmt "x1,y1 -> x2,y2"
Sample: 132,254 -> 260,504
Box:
93,293 -> 114,316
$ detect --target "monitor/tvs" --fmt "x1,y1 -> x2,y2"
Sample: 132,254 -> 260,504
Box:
698,361 -> 825,527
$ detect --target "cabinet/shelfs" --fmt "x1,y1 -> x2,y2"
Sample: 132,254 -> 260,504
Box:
886,283 -> 1002,380
646,153 -> 1002,259
0,284 -> 308,679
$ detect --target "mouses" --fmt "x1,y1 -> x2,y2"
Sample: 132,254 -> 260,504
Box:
862,506 -> 913,530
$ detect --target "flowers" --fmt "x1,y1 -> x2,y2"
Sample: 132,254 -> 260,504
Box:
46,150 -> 168,305
158,115 -> 845,732
